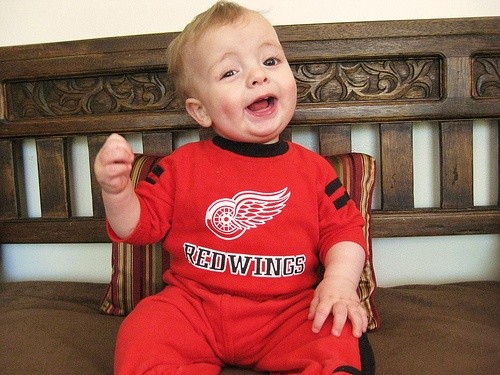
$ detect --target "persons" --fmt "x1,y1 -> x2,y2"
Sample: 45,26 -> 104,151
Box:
94,1 -> 368,375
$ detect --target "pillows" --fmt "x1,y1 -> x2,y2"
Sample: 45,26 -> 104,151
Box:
100,152 -> 387,332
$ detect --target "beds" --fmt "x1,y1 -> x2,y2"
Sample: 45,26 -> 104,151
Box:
0,16 -> 500,375
